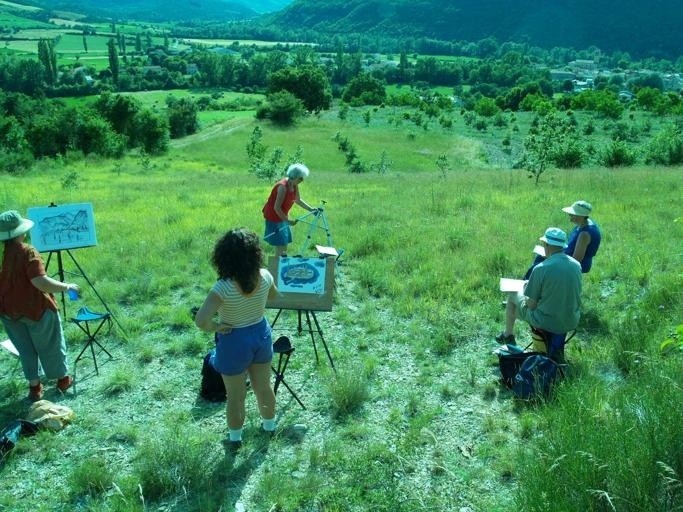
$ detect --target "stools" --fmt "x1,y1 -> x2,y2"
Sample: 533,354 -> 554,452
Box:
316,245 -> 345,263
267,334 -> 308,412
69,306 -> 114,376
523,319 -> 577,382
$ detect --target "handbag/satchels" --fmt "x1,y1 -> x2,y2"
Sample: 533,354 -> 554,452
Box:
201,352 -> 227,402
495,351 -> 564,400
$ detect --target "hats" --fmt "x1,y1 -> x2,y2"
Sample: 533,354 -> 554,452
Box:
0,210 -> 34,241
540,227 -> 568,249
562,201 -> 591,216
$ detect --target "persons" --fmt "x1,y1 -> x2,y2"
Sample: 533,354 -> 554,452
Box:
194,227 -> 283,450
0,210 -> 81,401
261,163 -> 310,255
495,227 -> 583,347
525,201 -> 601,279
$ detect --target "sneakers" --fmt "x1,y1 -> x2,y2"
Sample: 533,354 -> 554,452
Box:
496,332 -> 516,346
222,439 -> 241,449
259,424 -> 275,436
57,376 -> 72,392
28,383 -> 43,401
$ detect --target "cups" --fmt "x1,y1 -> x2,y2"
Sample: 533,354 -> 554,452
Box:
68,289 -> 79,301
